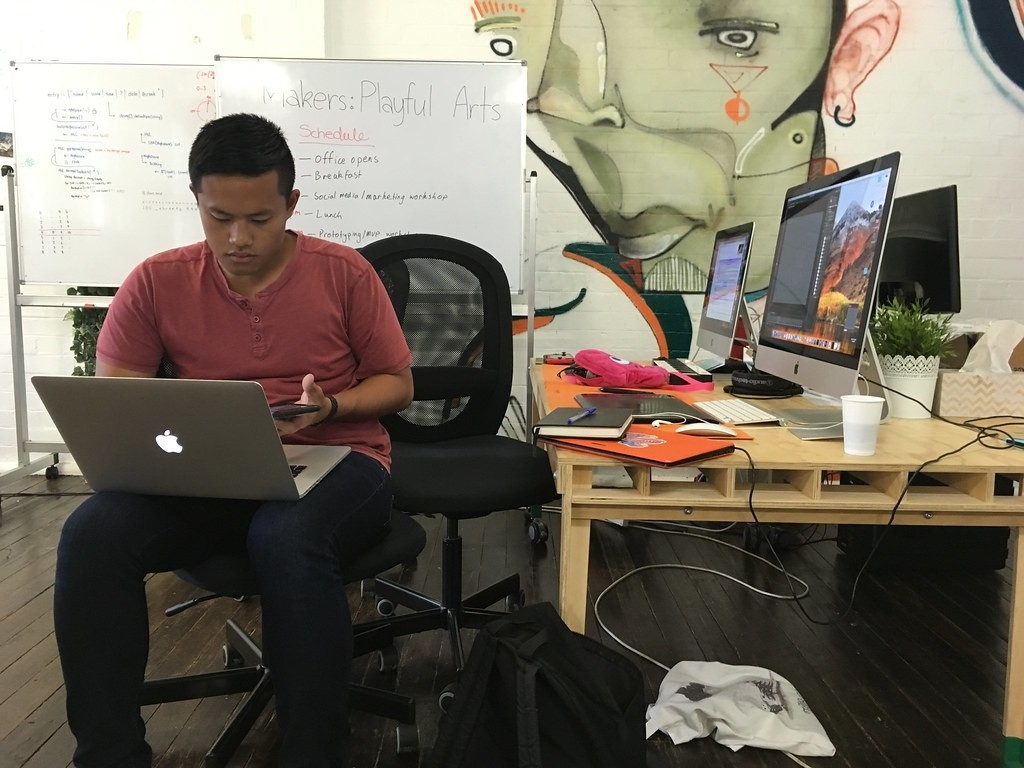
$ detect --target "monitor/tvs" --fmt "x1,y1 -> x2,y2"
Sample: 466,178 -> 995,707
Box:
877,184 -> 961,315
697,221 -> 759,361
755,151 -> 902,441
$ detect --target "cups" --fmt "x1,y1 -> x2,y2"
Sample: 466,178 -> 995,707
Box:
840,395 -> 885,456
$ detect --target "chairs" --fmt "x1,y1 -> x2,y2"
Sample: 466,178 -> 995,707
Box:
138,508 -> 427,768
335,234 -> 560,714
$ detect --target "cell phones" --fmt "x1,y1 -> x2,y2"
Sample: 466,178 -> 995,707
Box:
271,404 -> 321,418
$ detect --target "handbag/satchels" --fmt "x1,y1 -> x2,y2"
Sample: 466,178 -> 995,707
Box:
561,349 -> 669,388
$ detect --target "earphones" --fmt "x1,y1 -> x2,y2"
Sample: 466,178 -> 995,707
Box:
651,420 -> 673,428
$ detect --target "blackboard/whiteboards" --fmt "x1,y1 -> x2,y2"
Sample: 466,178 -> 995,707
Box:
6,55 -> 539,309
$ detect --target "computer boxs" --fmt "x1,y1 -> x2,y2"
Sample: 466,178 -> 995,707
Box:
836,525 -> 1011,575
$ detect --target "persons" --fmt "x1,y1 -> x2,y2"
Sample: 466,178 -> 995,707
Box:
54,113 -> 414,768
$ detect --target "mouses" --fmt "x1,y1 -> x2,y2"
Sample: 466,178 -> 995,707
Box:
676,423 -> 737,437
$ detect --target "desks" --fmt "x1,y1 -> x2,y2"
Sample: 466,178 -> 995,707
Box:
524,356 -> 1024,741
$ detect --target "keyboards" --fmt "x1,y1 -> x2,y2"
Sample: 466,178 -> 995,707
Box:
693,397 -> 788,427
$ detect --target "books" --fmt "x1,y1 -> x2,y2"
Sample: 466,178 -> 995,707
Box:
532,407 -> 635,441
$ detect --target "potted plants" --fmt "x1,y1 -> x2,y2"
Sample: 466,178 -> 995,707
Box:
867,294 -> 957,419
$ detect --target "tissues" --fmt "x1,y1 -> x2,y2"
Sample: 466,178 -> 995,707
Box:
933,320 -> 1024,417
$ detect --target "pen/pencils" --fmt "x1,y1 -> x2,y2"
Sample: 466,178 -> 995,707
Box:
566,407 -> 598,425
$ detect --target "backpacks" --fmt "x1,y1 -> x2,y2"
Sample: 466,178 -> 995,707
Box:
423,601 -> 648,768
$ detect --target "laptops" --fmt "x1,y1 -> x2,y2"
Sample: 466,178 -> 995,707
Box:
31,375 -> 351,500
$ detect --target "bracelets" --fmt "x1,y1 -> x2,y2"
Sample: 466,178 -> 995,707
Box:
311,395 -> 338,426
